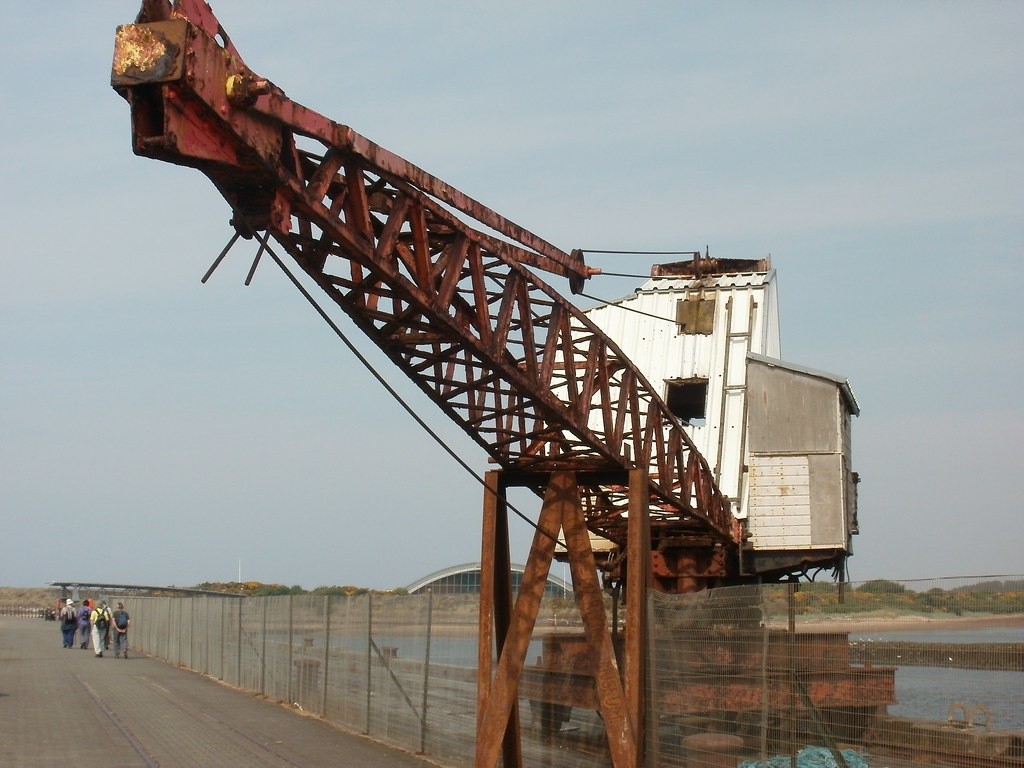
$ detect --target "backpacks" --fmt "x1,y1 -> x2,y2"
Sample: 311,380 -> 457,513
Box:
79,606 -> 90,620
94,608 -> 107,630
117,610 -> 127,629
65,606 -> 75,624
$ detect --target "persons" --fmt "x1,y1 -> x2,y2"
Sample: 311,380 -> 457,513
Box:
89,600 -> 110,657
111,602 -> 131,660
99,599 -> 114,651
59,598 -> 80,649
77,599 -> 93,650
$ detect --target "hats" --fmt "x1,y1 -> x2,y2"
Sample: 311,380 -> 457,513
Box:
65,598 -> 74,605
96,601 -> 102,608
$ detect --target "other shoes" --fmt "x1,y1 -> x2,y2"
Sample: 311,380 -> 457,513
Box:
124,651 -> 127,658
95,652 -> 103,657
